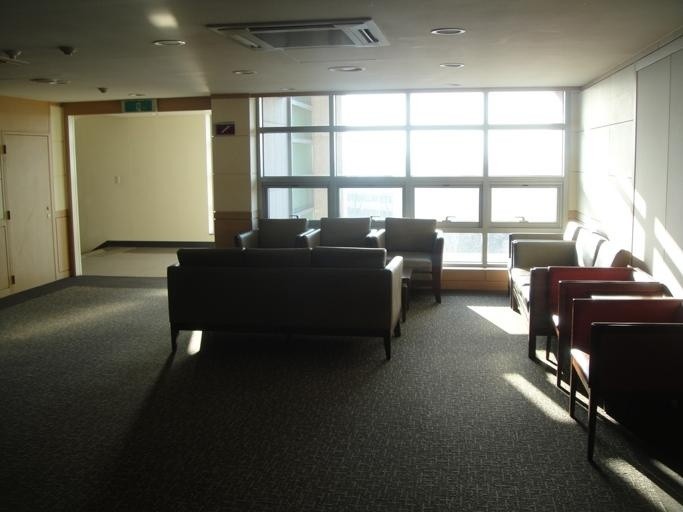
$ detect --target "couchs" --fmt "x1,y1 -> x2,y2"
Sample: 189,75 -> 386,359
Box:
570,298 -> 683,459
166,247 -> 403,359
508,221 -> 582,296
547,266 -> 659,386
373,218 -> 443,303
510,229 -> 630,319
303,219 -> 385,252
236,220 -> 305,252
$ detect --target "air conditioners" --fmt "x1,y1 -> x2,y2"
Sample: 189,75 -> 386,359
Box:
206,17 -> 391,53
